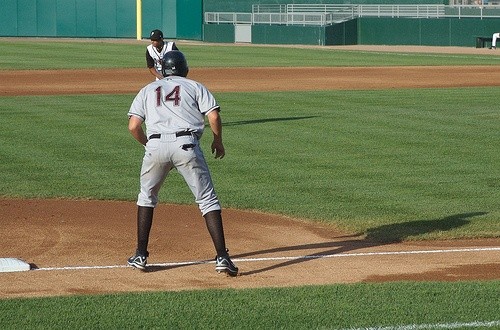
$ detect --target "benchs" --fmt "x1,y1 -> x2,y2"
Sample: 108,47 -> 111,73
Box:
475,36 -> 500,48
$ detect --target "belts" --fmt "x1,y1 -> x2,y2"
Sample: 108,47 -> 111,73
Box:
149,131 -> 191,140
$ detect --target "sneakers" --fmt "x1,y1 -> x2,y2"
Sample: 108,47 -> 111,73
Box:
216,248 -> 237,276
127,250 -> 150,269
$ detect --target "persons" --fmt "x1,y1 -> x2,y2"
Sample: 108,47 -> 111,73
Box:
489,32 -> 500,49
146,29 -> 179,80
126,51 -> 239,278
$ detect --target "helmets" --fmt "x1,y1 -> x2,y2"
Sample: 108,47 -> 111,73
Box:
162,50 -> 188,77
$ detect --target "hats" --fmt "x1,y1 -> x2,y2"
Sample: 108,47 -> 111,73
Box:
148,29 -> 163,39
